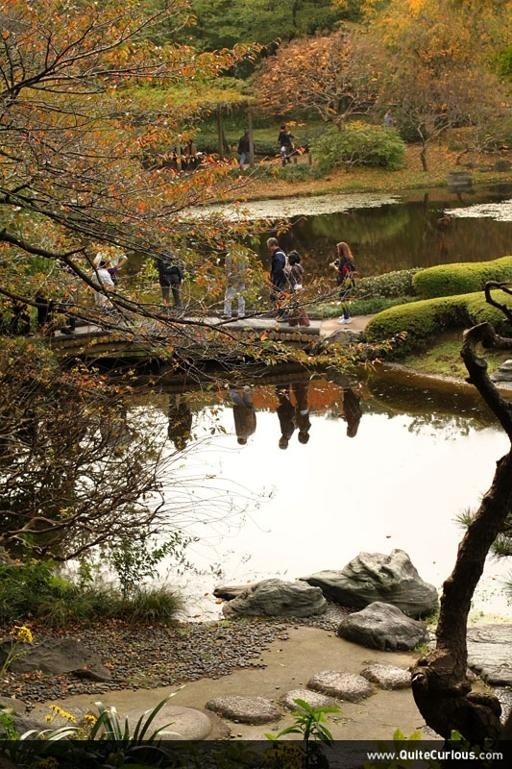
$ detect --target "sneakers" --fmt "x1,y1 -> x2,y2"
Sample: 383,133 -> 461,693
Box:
336,313 -> 353,324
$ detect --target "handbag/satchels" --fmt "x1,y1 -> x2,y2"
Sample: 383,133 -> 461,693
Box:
293,283 -> 303,290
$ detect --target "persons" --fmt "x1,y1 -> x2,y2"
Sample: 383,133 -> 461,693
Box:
277,124 -> 295,168
329,242 -> 356,324
274,384 -> 297,449
340,387 -> 362,438
385,109 -> 394,125
291,381 -> 312,445
88,254 -> 115,331
266,237 -> 288,323
100,397 -> 139,458
184,138 -> 199,170
227,386 -> 256,445
220,238 -> 246,319
167,391 -> 193,452
173,144 -> 187,168
109,243 -> 128,288
153,246 -> 184,319
237,130 -> 256,170
284,252 -> 310,327
